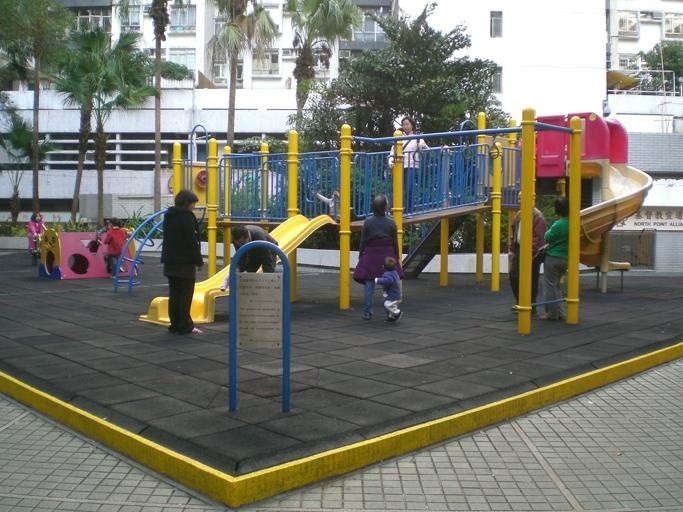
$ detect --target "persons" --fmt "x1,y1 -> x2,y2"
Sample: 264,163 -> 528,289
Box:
353,194 -> 403,322
229,222 -> 277,271
219,257 -> 247,292
309,184 -> 342,224
161,186 -> 204,335
103,214 -> 126,281
538,194 -> 569,322
28,209 -> 44,264
506,185 -> 548,313
372,251 -> 401,324
385,114 -> 431,215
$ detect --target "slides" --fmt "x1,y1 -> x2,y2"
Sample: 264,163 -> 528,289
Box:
580,166 -> 653,269
139,213 -> 337,326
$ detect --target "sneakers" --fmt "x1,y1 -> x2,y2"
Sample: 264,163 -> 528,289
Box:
384,311 -> 403,323
539,312 -> 558,320
189,327 -> 202,335
362,312 -> 373,320
